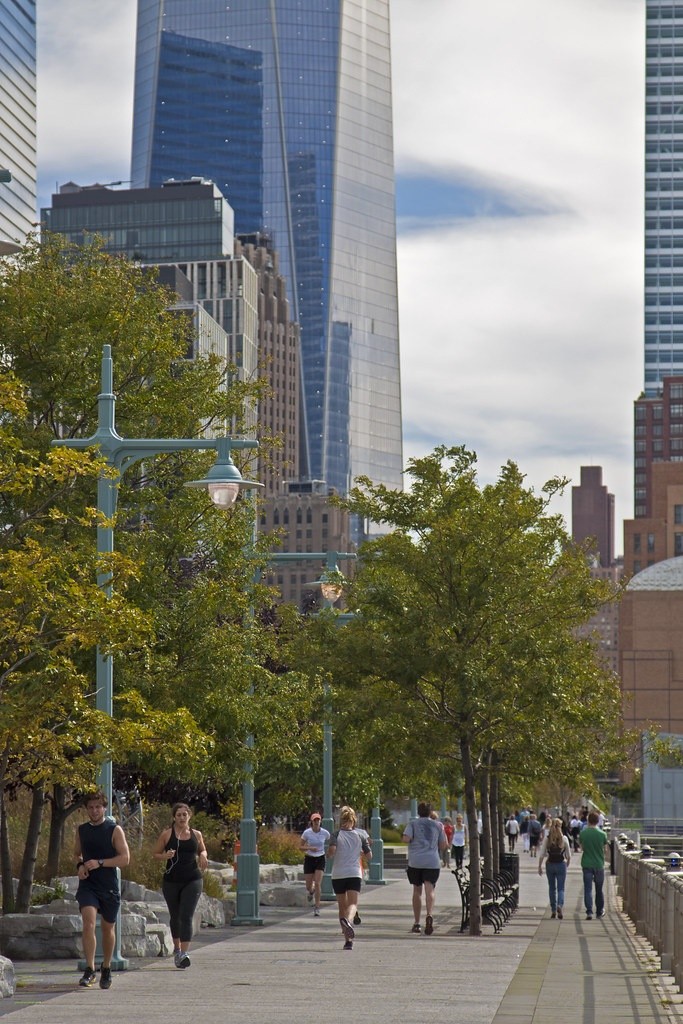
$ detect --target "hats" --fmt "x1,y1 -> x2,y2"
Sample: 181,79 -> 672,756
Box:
310,813 -> 321,821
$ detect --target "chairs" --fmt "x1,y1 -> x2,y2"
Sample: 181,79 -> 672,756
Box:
452,861 -> 519,934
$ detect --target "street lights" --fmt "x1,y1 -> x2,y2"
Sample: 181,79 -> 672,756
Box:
51,343 -> 267,975
207,489 -> 359,925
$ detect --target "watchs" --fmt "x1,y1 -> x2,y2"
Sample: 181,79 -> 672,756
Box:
98,859 -> 104,867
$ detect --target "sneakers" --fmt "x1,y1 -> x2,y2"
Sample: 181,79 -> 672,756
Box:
180,955 -> 190,968
340,917 -> 354,939
174,947 -> 181,967
79,967 -> 97,987
412,923 -> 421,932
100,962 -> 112,989
343,941 -> 353,949
308,887 -> 315,901
425,913 -> 433,935
314,906 -> 319,916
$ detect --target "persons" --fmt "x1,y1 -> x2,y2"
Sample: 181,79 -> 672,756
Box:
401,803 -> 446,934
579,812 -> 607,917
430,812 -> 467,868
538,817 -> 570,919
155,803 -> 207,969
326,806 -> 373,949
299,813 -> 330,916
505,808 -> 609,858
73,792 -> 130,992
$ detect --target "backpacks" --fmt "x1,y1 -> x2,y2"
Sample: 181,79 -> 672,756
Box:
546,835 -> 565,863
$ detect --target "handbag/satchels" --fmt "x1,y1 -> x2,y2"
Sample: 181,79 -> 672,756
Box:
532,828 -> 539,834
505,820 -> 510,835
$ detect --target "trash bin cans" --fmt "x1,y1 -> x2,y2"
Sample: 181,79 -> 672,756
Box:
505,853 -> 519,904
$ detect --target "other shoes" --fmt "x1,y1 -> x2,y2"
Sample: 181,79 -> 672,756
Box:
586,913 -> 592,920
556,905 -> 563,919
353,910 -> 361,924
596,908 -> 605,919
551,911 -> 556,919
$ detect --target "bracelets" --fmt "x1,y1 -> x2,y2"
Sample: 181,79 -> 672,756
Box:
76,862 -> 84,871
